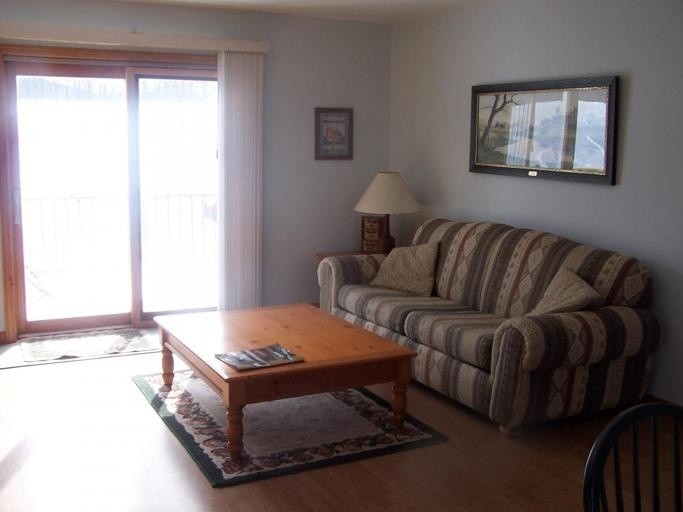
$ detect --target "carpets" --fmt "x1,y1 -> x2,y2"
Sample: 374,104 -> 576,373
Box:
130,372 -> 451,492
17,324 -> 161,364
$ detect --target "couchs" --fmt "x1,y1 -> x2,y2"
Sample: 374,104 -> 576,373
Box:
310,216 -> 660,437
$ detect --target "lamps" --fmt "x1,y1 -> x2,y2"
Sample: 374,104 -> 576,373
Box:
351,168 -> 423,257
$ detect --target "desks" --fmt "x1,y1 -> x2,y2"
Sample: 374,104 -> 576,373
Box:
312,248 -> 374,270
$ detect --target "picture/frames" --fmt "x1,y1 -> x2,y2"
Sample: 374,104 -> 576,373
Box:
469,70 -> 620,186
312,106 -> 355,161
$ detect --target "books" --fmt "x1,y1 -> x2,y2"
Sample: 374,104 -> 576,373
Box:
214,342 -> 304,372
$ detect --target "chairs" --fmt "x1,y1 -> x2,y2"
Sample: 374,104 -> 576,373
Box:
573,400 -> 680,512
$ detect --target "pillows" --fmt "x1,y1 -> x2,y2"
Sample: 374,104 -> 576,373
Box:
519,263 -> 603,319
369,239 -> 441,300
